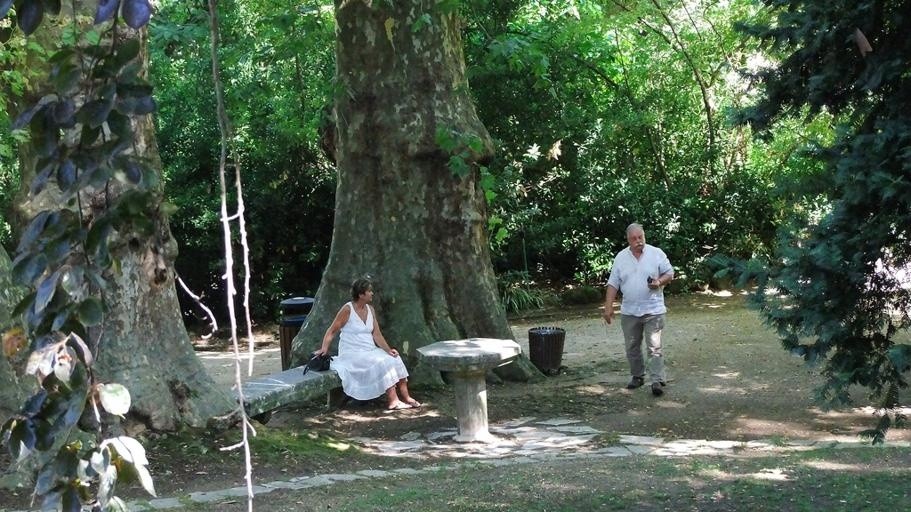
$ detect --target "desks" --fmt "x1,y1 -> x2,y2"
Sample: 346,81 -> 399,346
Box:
417,336 -> 522,441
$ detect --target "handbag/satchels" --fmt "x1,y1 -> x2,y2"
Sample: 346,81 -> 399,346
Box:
309,352 -> 330,371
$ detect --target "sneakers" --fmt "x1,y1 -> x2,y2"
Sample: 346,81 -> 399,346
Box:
652,382 -> 663,394
628,377 -> 643,388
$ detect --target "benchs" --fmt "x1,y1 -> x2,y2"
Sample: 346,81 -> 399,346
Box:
230,363 -> 341,418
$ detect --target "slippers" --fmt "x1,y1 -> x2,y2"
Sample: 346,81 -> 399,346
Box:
388,397 -> 421,409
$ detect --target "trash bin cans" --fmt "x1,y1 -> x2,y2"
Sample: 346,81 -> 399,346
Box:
274,297 -> 315,371
528,327 -> 566,377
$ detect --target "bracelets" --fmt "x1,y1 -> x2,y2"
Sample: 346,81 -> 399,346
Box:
657,279 -> 662,284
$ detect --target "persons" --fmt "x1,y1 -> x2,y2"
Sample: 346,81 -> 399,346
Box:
312,274 -> 421,410
603,222 -> 675,395
